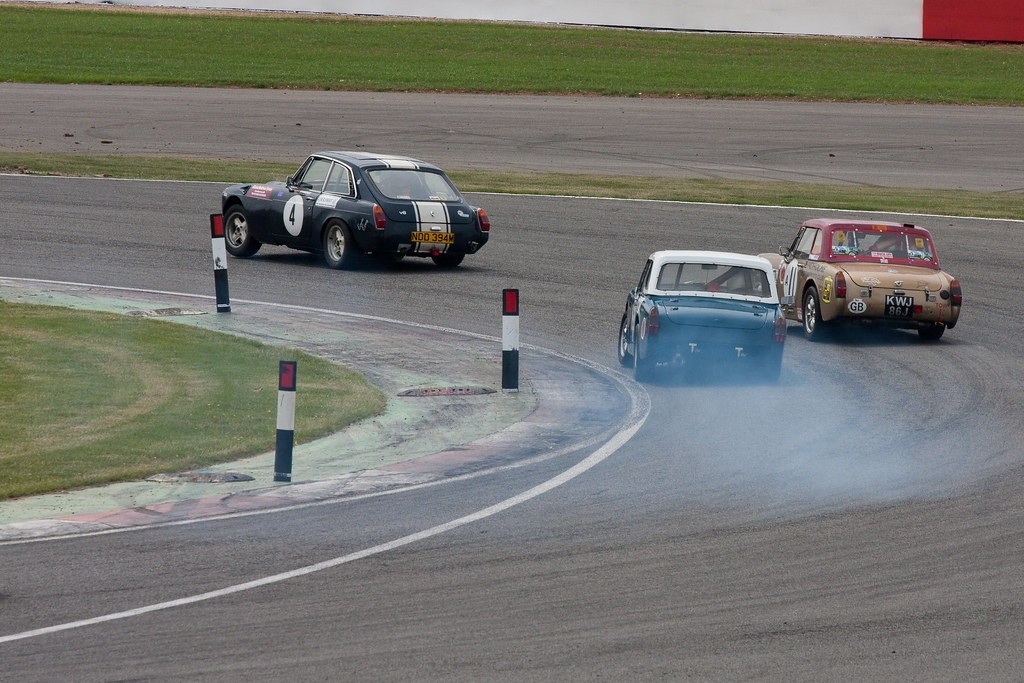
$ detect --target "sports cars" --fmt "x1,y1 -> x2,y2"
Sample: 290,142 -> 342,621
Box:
617,250 -> 786,380
215,147 -> 490,270
755,218 -> 963,341
